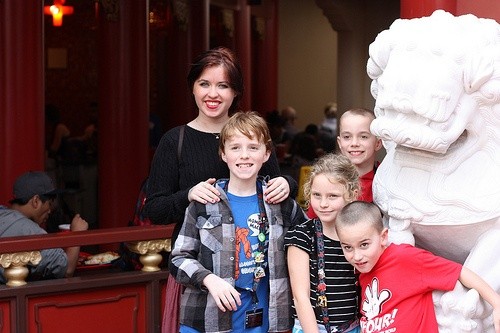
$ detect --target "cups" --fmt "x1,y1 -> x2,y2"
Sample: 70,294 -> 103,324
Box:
59,224 -> 70,231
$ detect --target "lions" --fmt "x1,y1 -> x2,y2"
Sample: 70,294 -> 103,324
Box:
367,8 -> 500,333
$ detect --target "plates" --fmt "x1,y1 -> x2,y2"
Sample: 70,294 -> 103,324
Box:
85,256 -> 121,265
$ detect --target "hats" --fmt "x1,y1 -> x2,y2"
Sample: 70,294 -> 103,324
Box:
12,169 -> 60,199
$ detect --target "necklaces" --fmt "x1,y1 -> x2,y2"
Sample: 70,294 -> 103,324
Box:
195,118 -> 221,140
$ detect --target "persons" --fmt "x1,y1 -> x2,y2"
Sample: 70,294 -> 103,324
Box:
144,48 -> 298,333
168,111 -> 312,333
263,103 -> 500,333
0,102 -> 98,280
